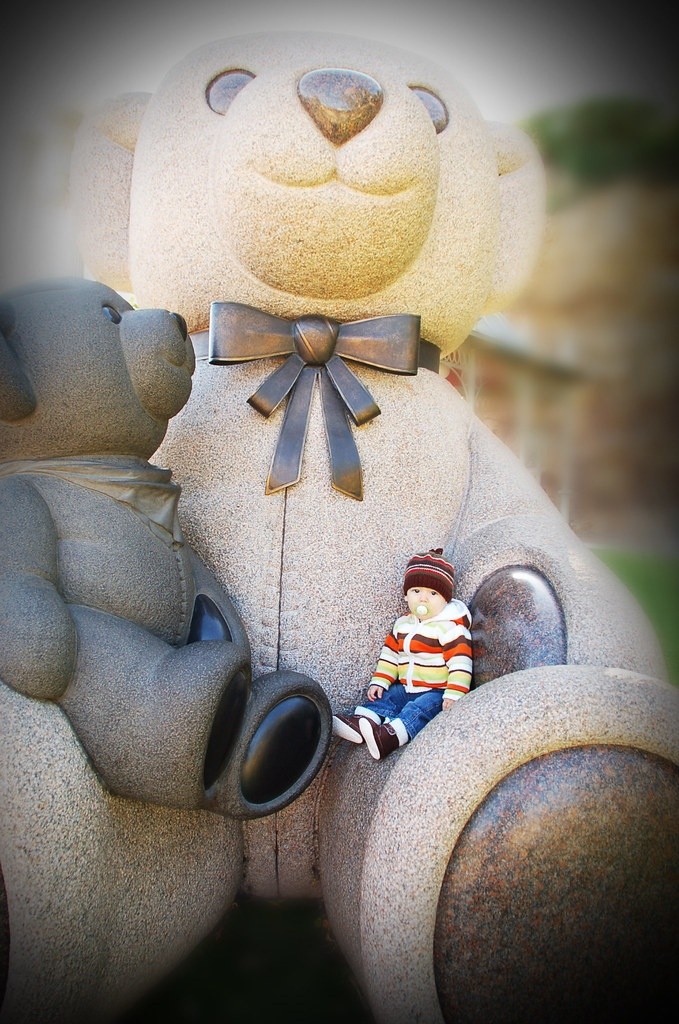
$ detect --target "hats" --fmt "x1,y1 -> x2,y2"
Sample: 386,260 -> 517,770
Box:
402,548 -> 455,603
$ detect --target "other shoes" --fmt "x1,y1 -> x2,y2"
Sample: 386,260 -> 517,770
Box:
332,705 -> 381,744
359,717 -> 408,760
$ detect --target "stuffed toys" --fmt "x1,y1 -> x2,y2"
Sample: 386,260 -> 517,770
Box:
0,277 -> 332,818
0,31 -> 677,1024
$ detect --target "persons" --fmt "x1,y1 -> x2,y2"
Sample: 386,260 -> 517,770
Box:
326,548 -> 473,762
